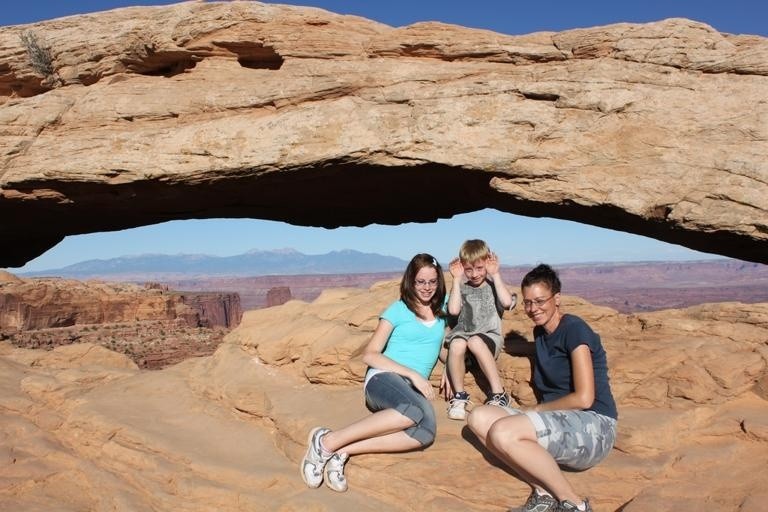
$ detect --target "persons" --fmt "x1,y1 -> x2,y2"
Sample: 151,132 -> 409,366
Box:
299,253 -> 453,492
467,264 -> 618,512
447,239 -> 518,421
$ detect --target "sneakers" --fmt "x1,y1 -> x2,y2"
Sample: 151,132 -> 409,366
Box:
446,391 -> 474,420
508,487 -> 592,512
485,387 -> 510,408
301,426 -> 348,492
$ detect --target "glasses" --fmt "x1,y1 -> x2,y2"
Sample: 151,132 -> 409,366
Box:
415,279 -> 438,286
521,295 -> 552,306
